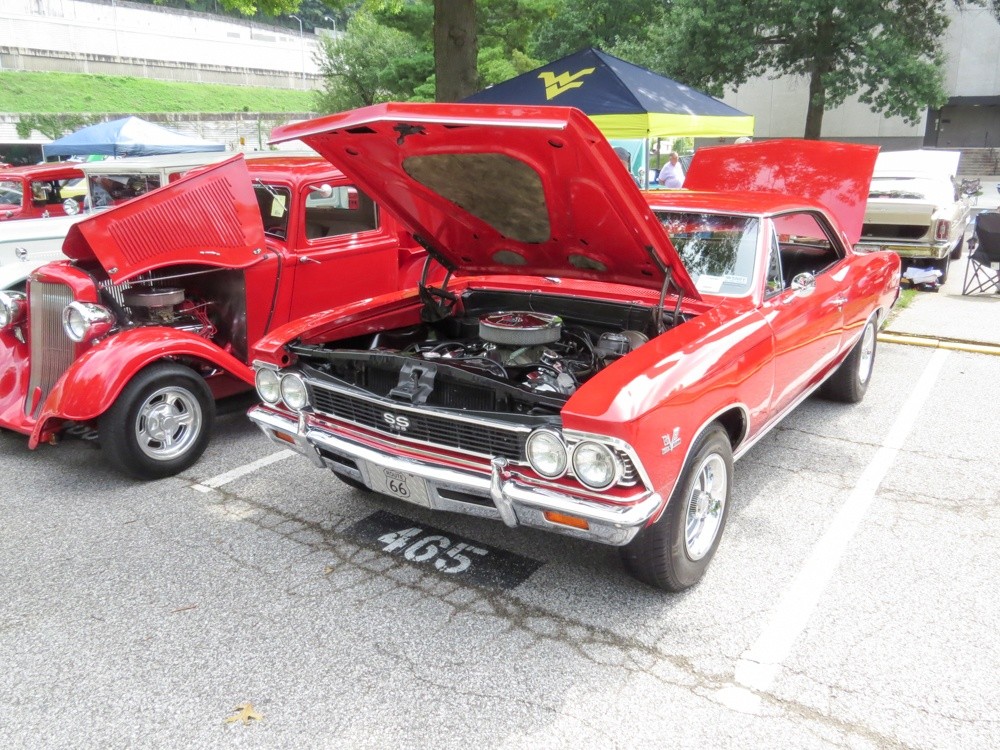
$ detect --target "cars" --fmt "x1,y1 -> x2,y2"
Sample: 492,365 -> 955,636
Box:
855,146 -> 975,284
243,98 -> 905,598
0,150 -> 471,480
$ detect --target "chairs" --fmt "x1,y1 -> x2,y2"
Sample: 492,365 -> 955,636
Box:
962,212 -> 1000,297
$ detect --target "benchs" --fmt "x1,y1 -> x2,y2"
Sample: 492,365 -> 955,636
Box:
302,218 -> 373,238
665,236 -> 833,285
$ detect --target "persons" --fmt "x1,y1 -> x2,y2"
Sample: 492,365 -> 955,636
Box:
658,151 -> 686,190
735,136 -> 752,144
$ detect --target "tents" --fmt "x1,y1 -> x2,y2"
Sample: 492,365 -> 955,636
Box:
461,47 -> 754,190
42,116 -> 226,163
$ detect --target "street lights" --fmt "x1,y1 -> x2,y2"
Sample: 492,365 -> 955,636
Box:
323,17 -> 336,43
288,15 -> 305,39
